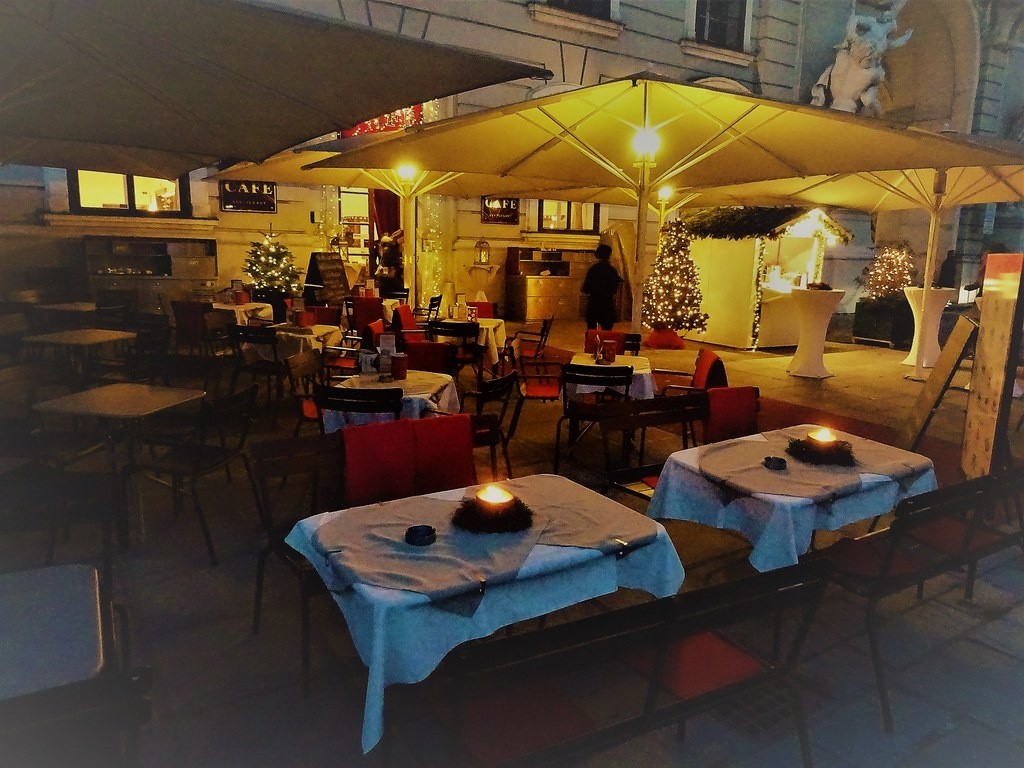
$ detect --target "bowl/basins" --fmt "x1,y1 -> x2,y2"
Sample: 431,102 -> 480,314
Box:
406,525 -> 435,545
764,456 -> 786,468
378,374 -> 393,383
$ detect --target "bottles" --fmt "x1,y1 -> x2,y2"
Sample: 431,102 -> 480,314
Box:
106,266 -> 169,276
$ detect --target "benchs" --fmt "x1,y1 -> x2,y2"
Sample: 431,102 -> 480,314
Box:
601,384 -> 762,505
779,464 -> 1024,738
245,410 -> 513,704
417,550 -> 841,766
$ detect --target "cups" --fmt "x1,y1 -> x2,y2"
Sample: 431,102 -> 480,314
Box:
390,353 -> 408,380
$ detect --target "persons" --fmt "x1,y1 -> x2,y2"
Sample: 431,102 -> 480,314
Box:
377,230 -> 403,273
583,244 -> 622,330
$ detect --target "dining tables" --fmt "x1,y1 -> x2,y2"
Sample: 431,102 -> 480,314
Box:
297,469 -> 688,756
563,353 -> 657,446
46,380 -> 209,533
1,562 -> 143,768
662,425 -> 940,676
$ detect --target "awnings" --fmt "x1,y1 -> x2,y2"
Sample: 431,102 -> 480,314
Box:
294,71 -> 1022,213
2,0 -> 554,182
201,126 -> 594,198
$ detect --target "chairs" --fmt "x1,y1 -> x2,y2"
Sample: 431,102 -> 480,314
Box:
554,362 -> 639,479
626,350 -> 729,468
585,329 -> 642,357
128,379 -> 264,563
2,283 -> 558,415
469,397 -> 524,480
0,419 -> 145,567
314,382 -> 404,427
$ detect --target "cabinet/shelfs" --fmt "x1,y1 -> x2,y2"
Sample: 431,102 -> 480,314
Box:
507,245 -> 575,320
